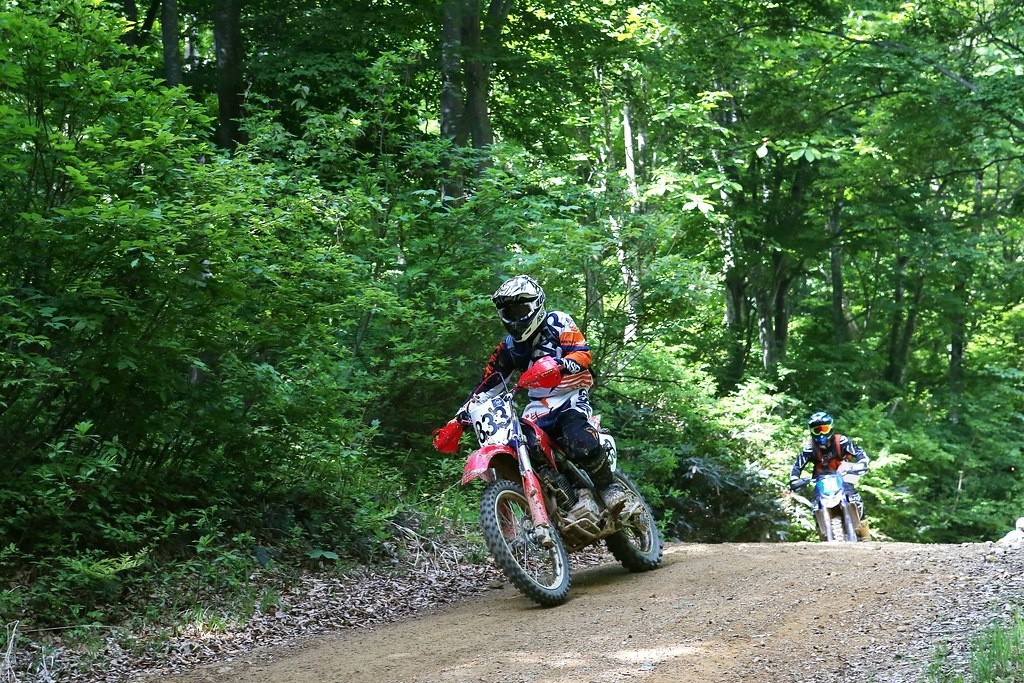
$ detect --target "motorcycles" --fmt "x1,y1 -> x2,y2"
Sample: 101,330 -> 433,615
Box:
788,467 -> 869,541
428,357 -> 667,604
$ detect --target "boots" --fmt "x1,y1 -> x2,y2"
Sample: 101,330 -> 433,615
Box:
815,526 -> 828,541
855,522 -> 872,541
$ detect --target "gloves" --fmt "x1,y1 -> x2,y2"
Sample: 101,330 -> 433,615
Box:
447,412 -> 471,432
554,357 -> 565,378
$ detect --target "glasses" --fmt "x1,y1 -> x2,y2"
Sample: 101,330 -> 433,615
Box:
496,288 -> 546,324
810,418 -> 834,436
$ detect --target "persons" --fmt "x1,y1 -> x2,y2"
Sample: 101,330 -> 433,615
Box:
790,412 -> 872,542
446,275 -> 629,512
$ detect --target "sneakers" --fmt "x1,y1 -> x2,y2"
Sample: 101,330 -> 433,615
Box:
598,483 -> 628,512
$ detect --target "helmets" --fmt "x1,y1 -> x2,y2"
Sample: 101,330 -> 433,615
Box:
492,275 -> 547,343
808,411 -> 835,446
